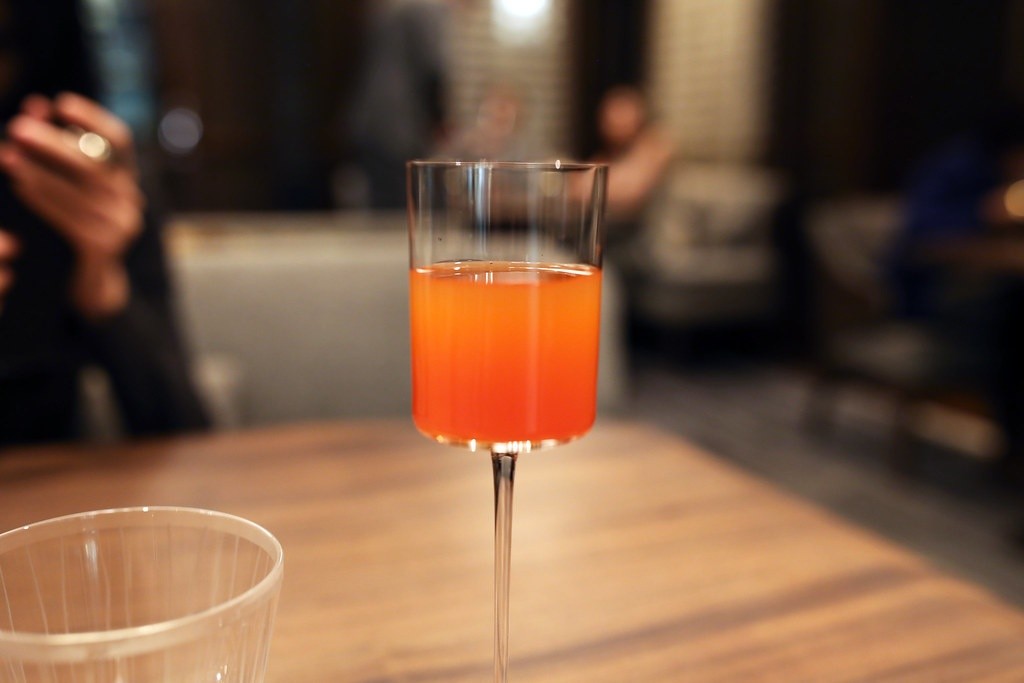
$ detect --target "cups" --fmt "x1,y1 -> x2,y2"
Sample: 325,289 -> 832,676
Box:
0,506 -> 287,683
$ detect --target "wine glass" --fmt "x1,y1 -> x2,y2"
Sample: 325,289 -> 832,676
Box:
408,159 -> 608,683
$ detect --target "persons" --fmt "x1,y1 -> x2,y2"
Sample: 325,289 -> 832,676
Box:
0,0 -> 213,446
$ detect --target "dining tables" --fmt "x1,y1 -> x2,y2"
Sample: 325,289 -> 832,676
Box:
0,416 -> 1023,682
